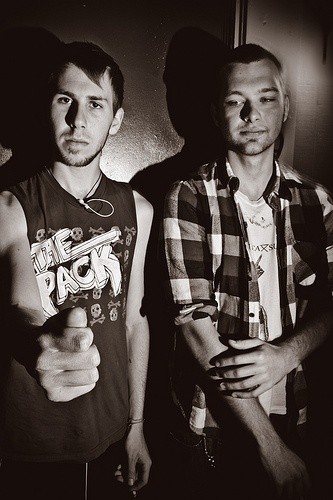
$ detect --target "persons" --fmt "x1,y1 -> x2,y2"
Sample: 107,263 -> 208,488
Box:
156,43 -> 332,500
0,42 -> 156,500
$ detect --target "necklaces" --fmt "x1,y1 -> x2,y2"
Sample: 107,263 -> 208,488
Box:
45,162 -> 102,199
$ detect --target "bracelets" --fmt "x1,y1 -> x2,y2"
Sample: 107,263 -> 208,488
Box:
128,418 -> 146,425
